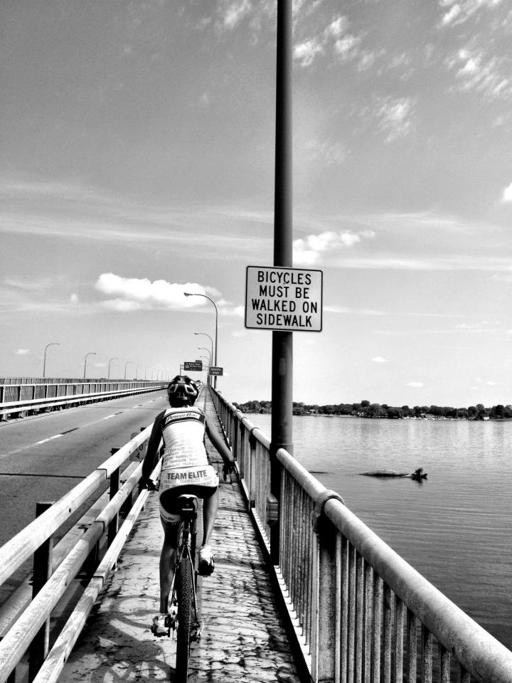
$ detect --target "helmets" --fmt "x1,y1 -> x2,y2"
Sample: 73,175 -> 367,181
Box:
167,376 -> 198,406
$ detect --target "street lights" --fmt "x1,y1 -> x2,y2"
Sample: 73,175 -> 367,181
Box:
183,291 -> 221,388
42,342 -> 60,379
106,357 -> 169,380
82,351 -> 96,378
193,329 -> 214,387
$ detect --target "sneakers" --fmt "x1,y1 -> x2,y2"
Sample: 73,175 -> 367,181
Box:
198,545 -> 214,570
153,615 -> 169,635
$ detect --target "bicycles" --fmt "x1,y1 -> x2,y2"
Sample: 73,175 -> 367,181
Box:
136,470 -> 234,683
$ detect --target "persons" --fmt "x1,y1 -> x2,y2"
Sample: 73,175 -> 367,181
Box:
142,374 -> 234,637
